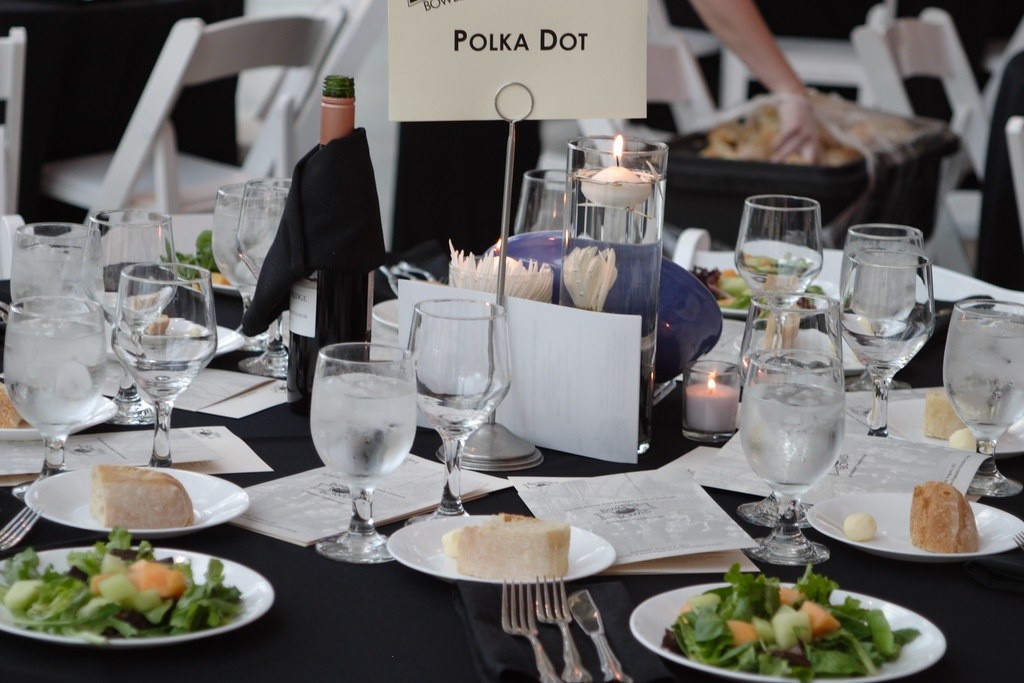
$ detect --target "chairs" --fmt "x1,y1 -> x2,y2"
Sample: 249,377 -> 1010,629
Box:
0,0 -> 1024,292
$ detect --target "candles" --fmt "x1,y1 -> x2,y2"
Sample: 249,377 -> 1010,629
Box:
582,135 -> 649,208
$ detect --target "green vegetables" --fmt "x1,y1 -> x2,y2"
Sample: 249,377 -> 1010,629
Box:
658,561 -> 921,683
695,253 -> 825,319
0,524 -> 246,646
160,229 -> 221,281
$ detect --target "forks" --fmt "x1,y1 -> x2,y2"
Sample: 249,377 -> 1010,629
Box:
0,506 -> 44,550
501,579 -> 563,683
536,574 -> 593,683
1013,531 -> 1024,550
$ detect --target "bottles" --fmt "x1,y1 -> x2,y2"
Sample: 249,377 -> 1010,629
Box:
286,74 -> 374,415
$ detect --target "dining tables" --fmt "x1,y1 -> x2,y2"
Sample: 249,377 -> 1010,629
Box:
0,213 -> 1024,683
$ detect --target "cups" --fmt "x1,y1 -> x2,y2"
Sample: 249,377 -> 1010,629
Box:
512,168 -> 575,235
680,359 -> 740,443
10,222 -> 105,314
557,131 -> 670,456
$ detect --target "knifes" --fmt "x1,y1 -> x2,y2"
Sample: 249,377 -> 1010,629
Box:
566,589 -> 633,683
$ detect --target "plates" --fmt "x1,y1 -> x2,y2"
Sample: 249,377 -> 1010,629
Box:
629,582 -> 947,683
386,514 -> 617,586
102,322 -> 246,359
686,246 -> 999,318
0,395 -> 121,440
806,490 -> 1024,564
23,465 -> 251,540
371,297 -> 398,332
0,545 -> 276,647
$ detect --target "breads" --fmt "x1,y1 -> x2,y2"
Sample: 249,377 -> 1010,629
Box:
145,315 -> 171,335
0,381 -> 32,429
700,102 -> 913,167
910,481 -> 980,554
923,392 -> 970,441
453,512 -> 571,581
91,465 -> 195,531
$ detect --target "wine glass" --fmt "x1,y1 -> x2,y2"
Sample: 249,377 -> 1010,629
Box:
210,177 -> 292,379
942,298 -> 1024,497
309,341 -> 419,565
734,195 -> 823,351
81,208 -> 181,426
111,262 -> 218,470
839,221 -> 926,399
737,293 -> 844,529
737,349 -> 847,566
4,296 -> 109,501
840,245 -> 936,439
404,298 -> 513,526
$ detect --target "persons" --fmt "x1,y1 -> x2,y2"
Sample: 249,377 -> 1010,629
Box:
690,0 -> 843,166
